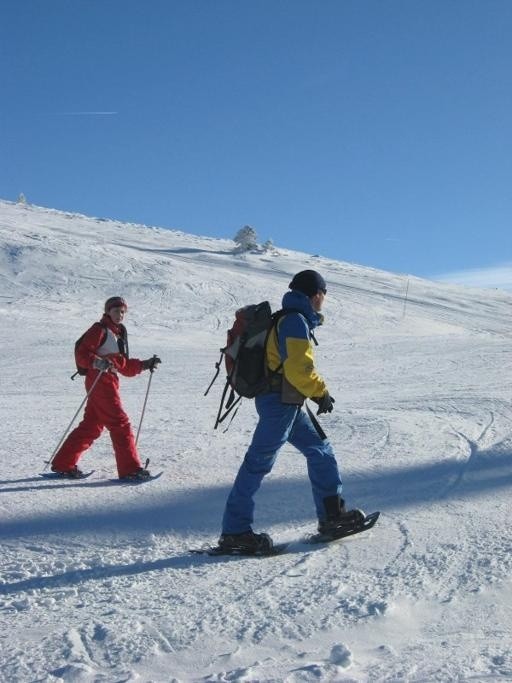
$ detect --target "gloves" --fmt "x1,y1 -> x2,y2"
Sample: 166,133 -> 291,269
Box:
143,357 -> 160,372
312,392 -> 335,415
95,358 -> 113,373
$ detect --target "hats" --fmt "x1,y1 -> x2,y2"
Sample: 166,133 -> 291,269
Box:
288,269 -> 326,299
105,297 -> 128,312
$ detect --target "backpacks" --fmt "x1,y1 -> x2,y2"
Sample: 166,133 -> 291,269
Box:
74,321 -> 108,376
224,299 -> 311,398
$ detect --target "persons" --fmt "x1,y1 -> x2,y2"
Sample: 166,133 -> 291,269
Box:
217,268 -> 369,549
50,295 -> 163,479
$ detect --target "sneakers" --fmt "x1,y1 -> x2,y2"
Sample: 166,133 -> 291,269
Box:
317,507 -> 366,535
120,467 -> 149,480
50,463 -> 81,477
217,531 -> 272,552
